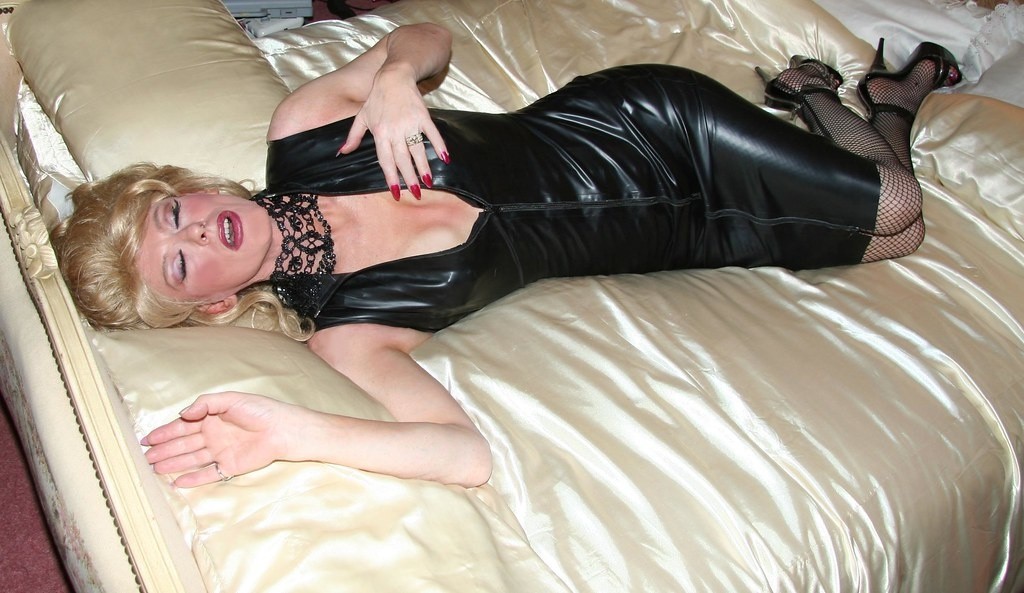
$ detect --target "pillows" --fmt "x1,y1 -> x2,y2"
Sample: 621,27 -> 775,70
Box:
9,2 -> 293,194
88,324 -> 572,593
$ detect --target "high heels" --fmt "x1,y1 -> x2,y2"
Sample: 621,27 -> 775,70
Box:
857,37 -> 962,123
755,54 -> 844,119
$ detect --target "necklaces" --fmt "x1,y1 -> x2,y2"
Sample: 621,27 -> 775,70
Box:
252,192 -> 337,333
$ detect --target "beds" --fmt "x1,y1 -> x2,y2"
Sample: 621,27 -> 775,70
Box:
1,0 -> 1024,593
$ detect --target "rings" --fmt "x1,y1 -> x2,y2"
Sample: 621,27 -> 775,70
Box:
406,133 -> 424,146
215,464 -> 234,482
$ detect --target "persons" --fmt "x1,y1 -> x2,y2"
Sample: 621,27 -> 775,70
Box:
45,21 -> 963,489
